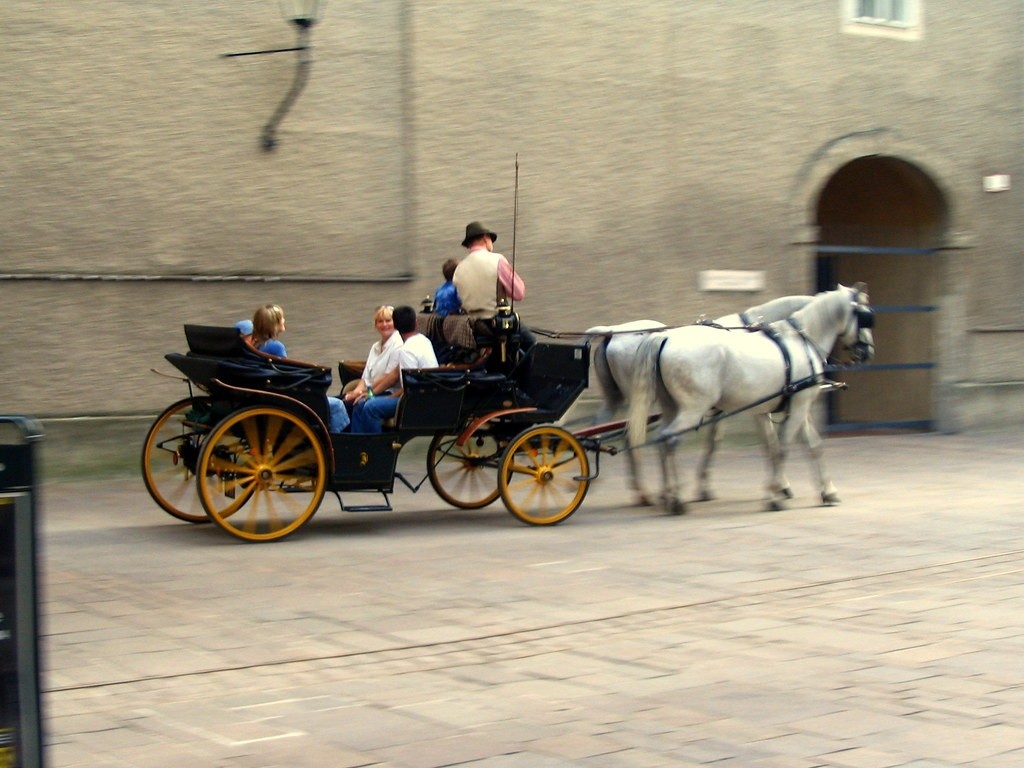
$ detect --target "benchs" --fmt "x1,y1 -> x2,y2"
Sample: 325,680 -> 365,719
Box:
184,324 -> 334,433
339,339 -> 491,429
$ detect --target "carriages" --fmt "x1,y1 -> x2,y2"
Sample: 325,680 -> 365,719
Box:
140,282 -> 875,543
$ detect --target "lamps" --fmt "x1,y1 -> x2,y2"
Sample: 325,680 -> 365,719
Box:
253,0 -> 325,148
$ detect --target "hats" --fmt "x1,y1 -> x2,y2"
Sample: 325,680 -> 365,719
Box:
461,221 -> 497,247
234,320 -> 254,335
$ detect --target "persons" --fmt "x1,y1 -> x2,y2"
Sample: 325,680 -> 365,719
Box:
433,259 -> 464,315
344,305 -> 440,434
234,304 -> 350,434
452,221 -> 537,355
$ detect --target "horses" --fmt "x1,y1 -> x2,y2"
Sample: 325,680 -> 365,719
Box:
577,283 -> 875,516
